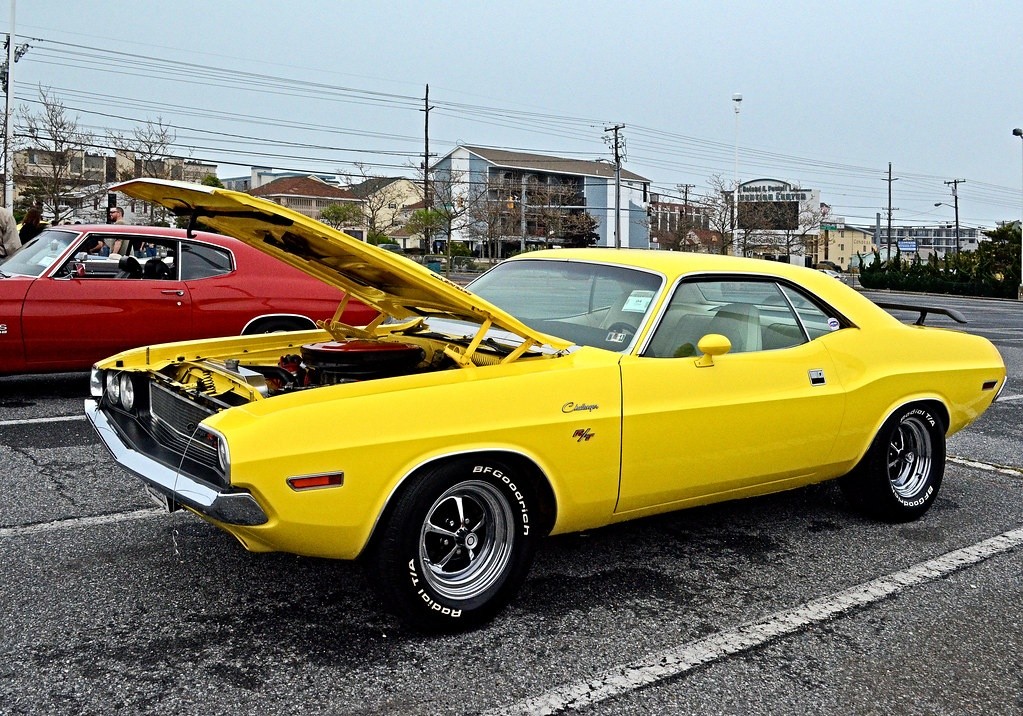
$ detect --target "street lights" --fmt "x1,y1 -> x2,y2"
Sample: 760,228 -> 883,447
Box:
595,123 -> 625,248
934,179 -> 966,254
732,93 -> 743,255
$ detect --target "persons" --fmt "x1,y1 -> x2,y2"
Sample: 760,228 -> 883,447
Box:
132,223 -> 157,258
0,207 -> 22,259
89,237 -> 105,253
19,208 -> 45,245
109,206 -> 133,255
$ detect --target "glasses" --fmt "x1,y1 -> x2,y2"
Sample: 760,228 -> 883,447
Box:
109,211 -> 119,214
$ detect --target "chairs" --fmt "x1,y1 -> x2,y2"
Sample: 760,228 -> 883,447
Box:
597,287 -> 763,356
113,256 -> 169,280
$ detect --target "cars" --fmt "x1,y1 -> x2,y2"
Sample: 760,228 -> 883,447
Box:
81,178 -> 1008,637
0,222 -> 416,380
